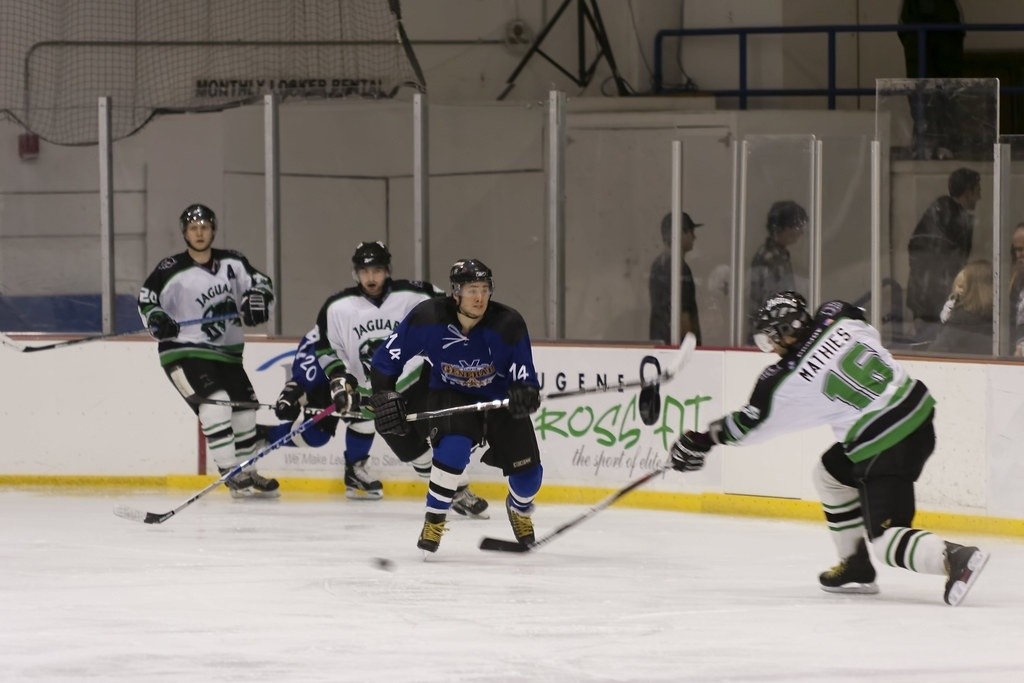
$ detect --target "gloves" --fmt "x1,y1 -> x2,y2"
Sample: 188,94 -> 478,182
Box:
240,286 -> 273,327
148,312 -> 180,339
329,373 -> 361,421
670,432 -> 714,472
277,381 -> 304,419
507,386 -> 540,418
370,389 -> 409,436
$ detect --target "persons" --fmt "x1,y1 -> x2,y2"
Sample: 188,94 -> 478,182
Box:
669,291 -> 980,604
316,241 -> 487,517
648,212 -> 705,346
137,204 -> 280,492
743,200 -> 808,347
371,257 -> 544,554
906,168 -> 1024,363
258,324 -> 384,491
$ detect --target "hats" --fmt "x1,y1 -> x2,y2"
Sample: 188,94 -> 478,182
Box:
661,212 -> 704,236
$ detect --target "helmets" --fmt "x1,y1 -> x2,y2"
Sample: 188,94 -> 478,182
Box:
353,241 -> 390,271
181,205 -> 215,233
450,259 -> 493,293
754,291 -> 811,342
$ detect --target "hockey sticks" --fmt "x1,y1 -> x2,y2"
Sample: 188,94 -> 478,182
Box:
0,311 -> 244,353
112,402 -> 337,525
479,463 -> 672,553
170,363 -> 369,421
405,331 -> 699,422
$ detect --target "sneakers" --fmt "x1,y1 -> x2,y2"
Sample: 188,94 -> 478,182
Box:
344,451 -> 382,499
452,490 -> 490,519
219,466 -> 257,497
506,492 -> 535,546
414,458 -> 435,481
417,512 -> 449,562
819,538 -> 878,595
244,471 -> 279,497
943,541 -> 991,605
